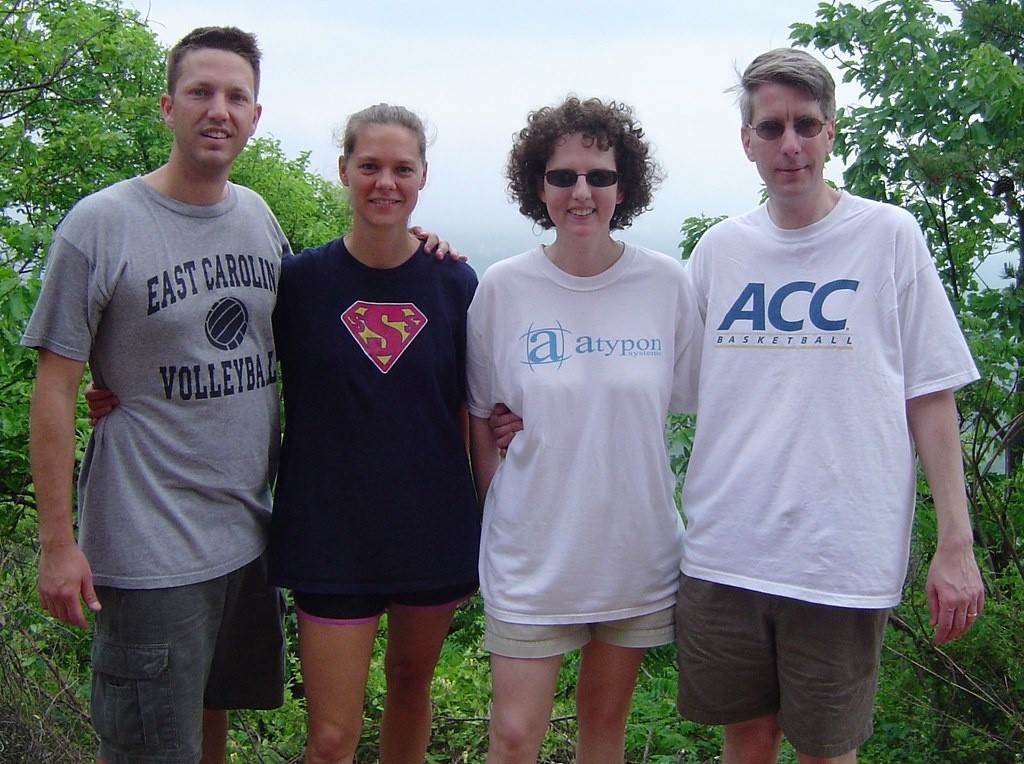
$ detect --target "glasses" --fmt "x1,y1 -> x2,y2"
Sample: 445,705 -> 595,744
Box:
543,168 -> 619,188
749,118 -> 827,140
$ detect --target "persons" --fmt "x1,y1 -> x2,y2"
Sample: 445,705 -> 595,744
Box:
20,27 -> 467,764
84,104 -> 478,763
466,98 -> 703,763
489,48 -> 984,764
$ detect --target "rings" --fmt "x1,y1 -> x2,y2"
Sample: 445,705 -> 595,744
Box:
967,614 -> 978,617
